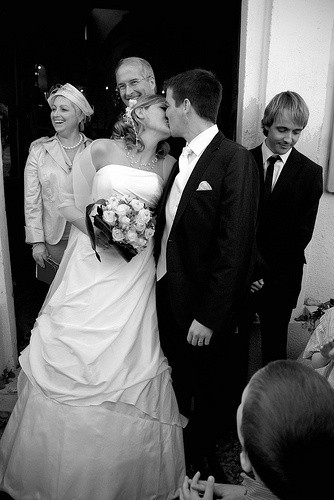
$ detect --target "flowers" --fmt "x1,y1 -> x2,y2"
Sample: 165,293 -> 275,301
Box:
124,99 -> 137,118
93,194 -> 156,263
295,297 -> 334,331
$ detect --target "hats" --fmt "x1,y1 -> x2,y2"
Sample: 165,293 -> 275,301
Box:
47,83 -> 94,116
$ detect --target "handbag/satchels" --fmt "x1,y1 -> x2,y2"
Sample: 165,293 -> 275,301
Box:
35,254 -> 61,284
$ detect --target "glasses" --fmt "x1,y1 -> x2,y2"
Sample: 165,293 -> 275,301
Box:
117,76 -> 151,90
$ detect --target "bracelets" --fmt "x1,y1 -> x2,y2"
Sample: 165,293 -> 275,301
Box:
31,242 -> 42,250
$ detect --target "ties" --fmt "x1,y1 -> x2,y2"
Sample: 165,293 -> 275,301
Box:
155,146 -> 193,283
265,155 -> 281,198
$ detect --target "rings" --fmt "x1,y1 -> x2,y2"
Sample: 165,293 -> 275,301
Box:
102,242 -> 108,249
198,340 -> 203,344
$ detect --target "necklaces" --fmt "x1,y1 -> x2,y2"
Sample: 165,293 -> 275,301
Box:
120,134 -> 158,170
55,133 -> 82,150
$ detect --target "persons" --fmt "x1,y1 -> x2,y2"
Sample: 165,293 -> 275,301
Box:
303,306 -> 334,388
115,57 -> 157,108
179,359 -> 334,500
153,68 -> 261,498
245,90 -> 324,365
0,95 -> 178,500
22,84 -> 95,329
